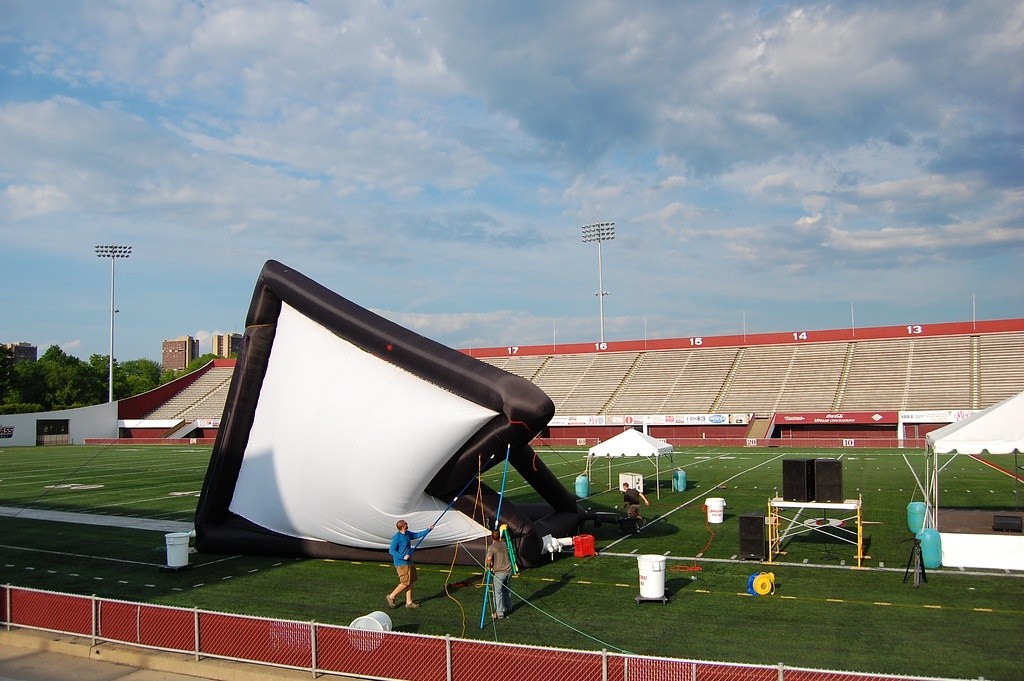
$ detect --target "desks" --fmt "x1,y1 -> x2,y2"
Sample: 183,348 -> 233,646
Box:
767,497 -> 862,568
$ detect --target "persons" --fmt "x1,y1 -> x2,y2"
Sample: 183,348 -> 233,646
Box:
486,529 -> 514,619
386,519 -> 436,609
621,482 -> 651,532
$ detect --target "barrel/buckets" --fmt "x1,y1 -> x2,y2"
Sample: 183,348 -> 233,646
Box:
674,469 -> 686,491
348,611 -> 392,651
165,532 -> 189,567
575,476 -> 588,498
917,529 -> 941,568
637,555 -> 666,599
705,498 -> 725,523
907,502 -> 926,533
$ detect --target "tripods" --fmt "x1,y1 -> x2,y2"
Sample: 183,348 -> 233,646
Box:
896,537 -> 928,589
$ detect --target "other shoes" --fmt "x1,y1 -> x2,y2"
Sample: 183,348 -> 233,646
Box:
385,595 -> 396,609
405,601 -> 420,608
642,518 -> 646,526
635,527 -> 640,532
491,613 -> 500,619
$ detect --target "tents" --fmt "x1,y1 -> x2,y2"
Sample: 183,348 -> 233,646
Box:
588,428 -> 675,497
924,391 -> 1024,533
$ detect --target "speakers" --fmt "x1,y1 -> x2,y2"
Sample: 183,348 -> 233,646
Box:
992,515 -> 1023,533
782,458 -> 816,502
815,459 -> 844,503
739,512 -> 769,562
